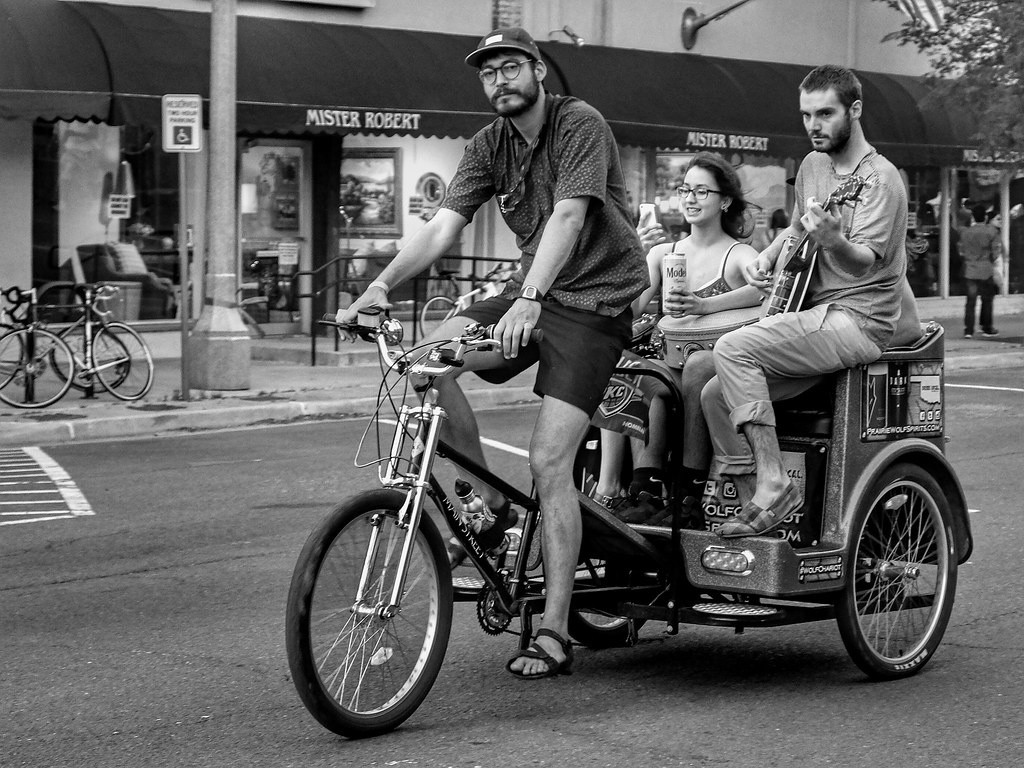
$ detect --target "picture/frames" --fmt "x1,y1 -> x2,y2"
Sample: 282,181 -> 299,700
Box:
275,155 -> 300,189
269,190 -> 299,231
336,146 -> 403,240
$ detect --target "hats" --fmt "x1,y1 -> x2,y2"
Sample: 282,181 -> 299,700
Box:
464,28 -> 542,60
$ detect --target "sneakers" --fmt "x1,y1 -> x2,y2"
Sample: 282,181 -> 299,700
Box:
606,485 -> 664,523
965,328 -> 974,338
642,491 -> 706,531
982,328 -> 999,338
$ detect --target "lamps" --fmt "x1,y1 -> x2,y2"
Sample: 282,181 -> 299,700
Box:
564,25 -> 586,49
240,183 -> 258,243
770,209 -> 791,229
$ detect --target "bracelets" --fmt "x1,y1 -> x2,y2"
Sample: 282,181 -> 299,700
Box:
368,282 -> 390,294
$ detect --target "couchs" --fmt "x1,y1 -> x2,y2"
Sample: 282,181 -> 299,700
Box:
77,243 -> 173,322
336,248 -> 431,302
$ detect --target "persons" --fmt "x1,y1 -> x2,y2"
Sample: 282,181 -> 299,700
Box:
335,25 -> 650,679
589,64 -> 909,522
956,204 -> 1002,339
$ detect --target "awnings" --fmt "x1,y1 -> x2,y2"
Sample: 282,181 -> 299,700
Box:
0,0 -> 1024,172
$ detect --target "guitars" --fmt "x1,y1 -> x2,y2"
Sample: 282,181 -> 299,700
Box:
759,174 -> 872,321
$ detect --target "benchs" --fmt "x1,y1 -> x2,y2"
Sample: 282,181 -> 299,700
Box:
771,318 -> 944,437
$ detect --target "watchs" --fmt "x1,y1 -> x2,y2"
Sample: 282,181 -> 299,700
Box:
517,285 -> 544,304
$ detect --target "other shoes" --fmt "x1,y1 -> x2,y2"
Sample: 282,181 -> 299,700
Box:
715,481 -> 804,537
593,492 -> 621,513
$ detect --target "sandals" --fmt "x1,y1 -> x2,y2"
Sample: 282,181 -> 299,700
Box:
505,629 -> 573,679
444,496 -> 519,569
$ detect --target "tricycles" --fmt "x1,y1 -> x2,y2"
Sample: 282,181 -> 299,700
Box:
283,299 -> 976,741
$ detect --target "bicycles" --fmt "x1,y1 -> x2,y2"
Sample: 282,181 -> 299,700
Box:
0,284 -> 157,412
420,259 -> 521,337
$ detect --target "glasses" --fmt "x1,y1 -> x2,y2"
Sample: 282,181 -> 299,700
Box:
677,187 -> 721,199
475,59 -> 532,85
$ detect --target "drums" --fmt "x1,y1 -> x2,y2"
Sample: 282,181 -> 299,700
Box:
630,313 -> 659,360
657,306 -> 759,370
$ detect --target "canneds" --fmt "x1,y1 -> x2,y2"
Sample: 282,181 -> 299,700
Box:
662,252 -> 687,315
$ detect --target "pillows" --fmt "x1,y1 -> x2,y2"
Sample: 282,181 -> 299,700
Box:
107,241 -> 148,274
346,240 -> 374,279
375,242 -> 398,254
